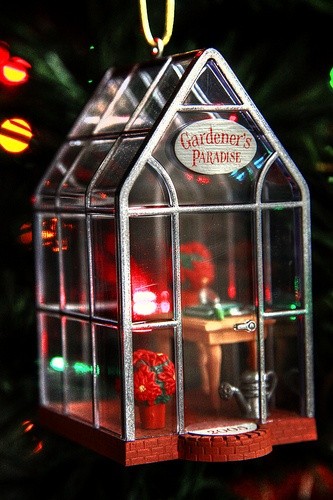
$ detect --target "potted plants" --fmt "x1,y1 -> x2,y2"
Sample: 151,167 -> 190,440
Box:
119,348 -> 178,429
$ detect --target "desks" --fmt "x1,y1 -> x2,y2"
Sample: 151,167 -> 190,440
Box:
144,308 -> 271,413
